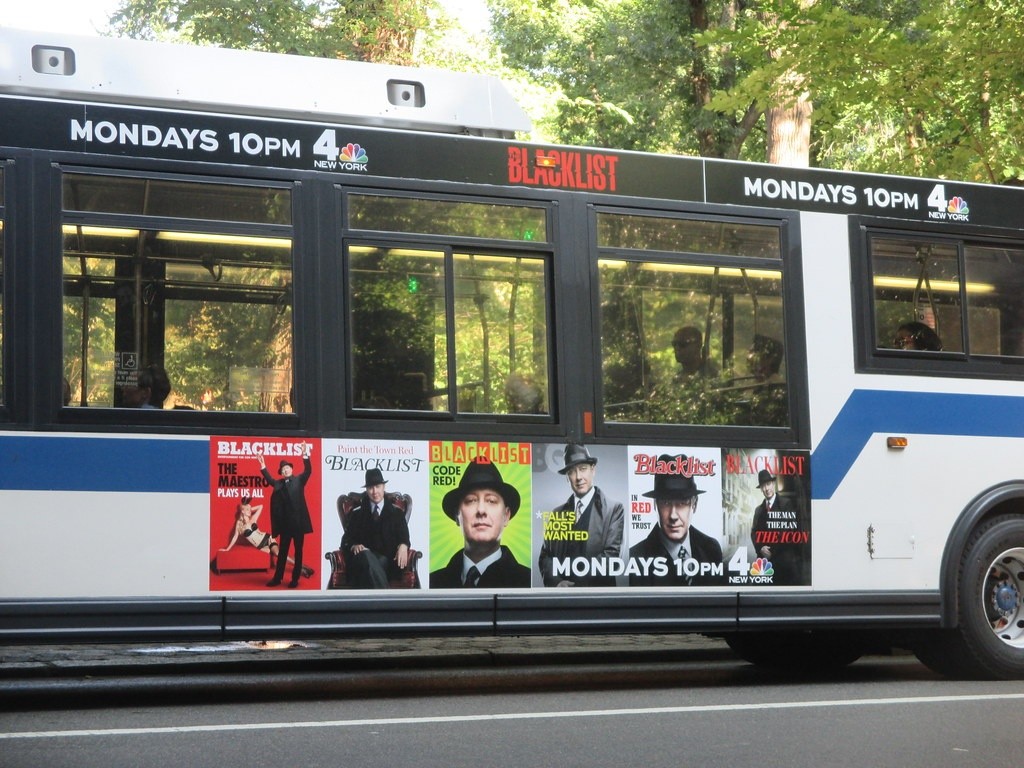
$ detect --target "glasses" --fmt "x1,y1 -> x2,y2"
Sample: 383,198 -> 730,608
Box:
892,336 -> 918,350
672,336 -> 699,348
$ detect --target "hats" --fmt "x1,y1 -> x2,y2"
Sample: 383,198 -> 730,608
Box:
755,470 -> 776,488
361,468 -> 389,487
442,457 -> 521,521
642,454 -> 707,499
557,441 -> 598,475
278,460 -> 293,475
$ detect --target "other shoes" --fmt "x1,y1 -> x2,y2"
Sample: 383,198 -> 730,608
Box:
267,578 -> 281,586
303,568 -> 313,577
288,580 -> 298,588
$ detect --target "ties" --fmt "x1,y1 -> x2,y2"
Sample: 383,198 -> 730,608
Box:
463,566 -> 480,588
575,500 -> 583,526
676,547 -> 687,574
767,501 -> 771,513
285,481 -> 290,487
371,505 -> 380,521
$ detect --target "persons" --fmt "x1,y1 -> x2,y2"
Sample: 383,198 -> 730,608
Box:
710,335 -> 781,423
340,468 -> 410,589
429,457 -> 531,589
628,455 -> 722,584
600,301 -> 648,411
217,504 -> 313,577
751,470 -> 803,585
352,299 -> 434,411
257,440 -> 311,588
538,443 -> 624,587
897,323 -> 943,352
670,326 -> 716,388
505,371 -> 546,412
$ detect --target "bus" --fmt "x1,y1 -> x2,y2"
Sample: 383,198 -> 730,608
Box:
0,20 -> 1024,682
